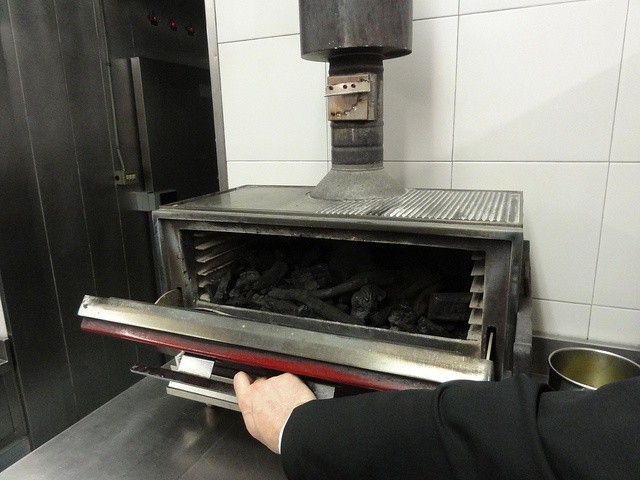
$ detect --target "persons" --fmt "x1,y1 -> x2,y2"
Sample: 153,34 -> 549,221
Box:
233,370 -> 640,480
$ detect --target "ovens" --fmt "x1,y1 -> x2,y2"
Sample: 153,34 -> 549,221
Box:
79,183 -> 534,414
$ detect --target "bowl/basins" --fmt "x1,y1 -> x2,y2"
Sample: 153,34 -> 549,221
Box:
548,346 -> 640,401
